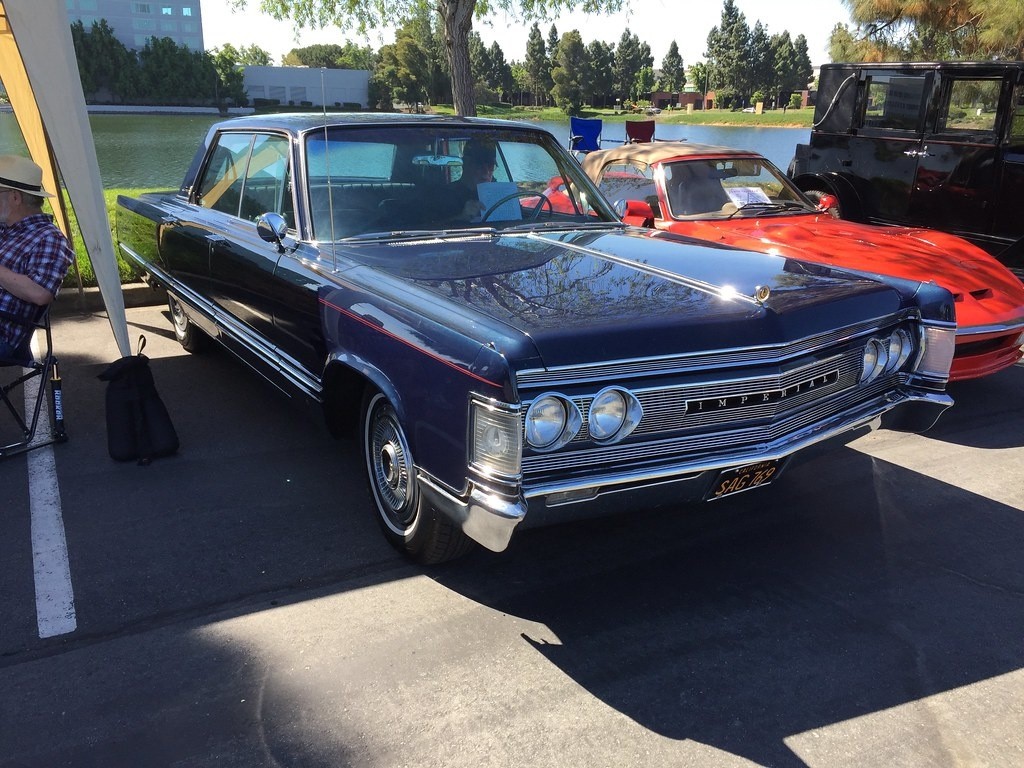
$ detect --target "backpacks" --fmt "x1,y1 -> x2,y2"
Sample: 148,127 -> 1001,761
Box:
96,334 -> 179,462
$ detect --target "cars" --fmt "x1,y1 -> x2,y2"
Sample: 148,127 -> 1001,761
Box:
776,59 -> 1024,283
115,112 -> 928,564
517,142 -> 1024,381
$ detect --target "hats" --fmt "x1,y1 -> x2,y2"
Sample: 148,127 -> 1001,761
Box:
0,155 -> 55,197
463,137 -> 498,167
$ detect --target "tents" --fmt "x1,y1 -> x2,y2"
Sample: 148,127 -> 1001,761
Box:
0,0 -> 131,357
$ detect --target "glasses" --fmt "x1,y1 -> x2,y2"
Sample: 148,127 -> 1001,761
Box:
472,162 -> 498,170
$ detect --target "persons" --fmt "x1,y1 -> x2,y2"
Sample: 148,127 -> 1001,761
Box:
0,155 -> 76,360
419,138 -> 520,225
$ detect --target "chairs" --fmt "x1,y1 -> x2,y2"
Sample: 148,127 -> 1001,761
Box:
0,294 -> 67,459
565,116 -> 602,158
625,120 -> 688,148
677,162 -> 727,213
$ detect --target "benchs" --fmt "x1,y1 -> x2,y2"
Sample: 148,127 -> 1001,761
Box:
244,181 -> 417,221
